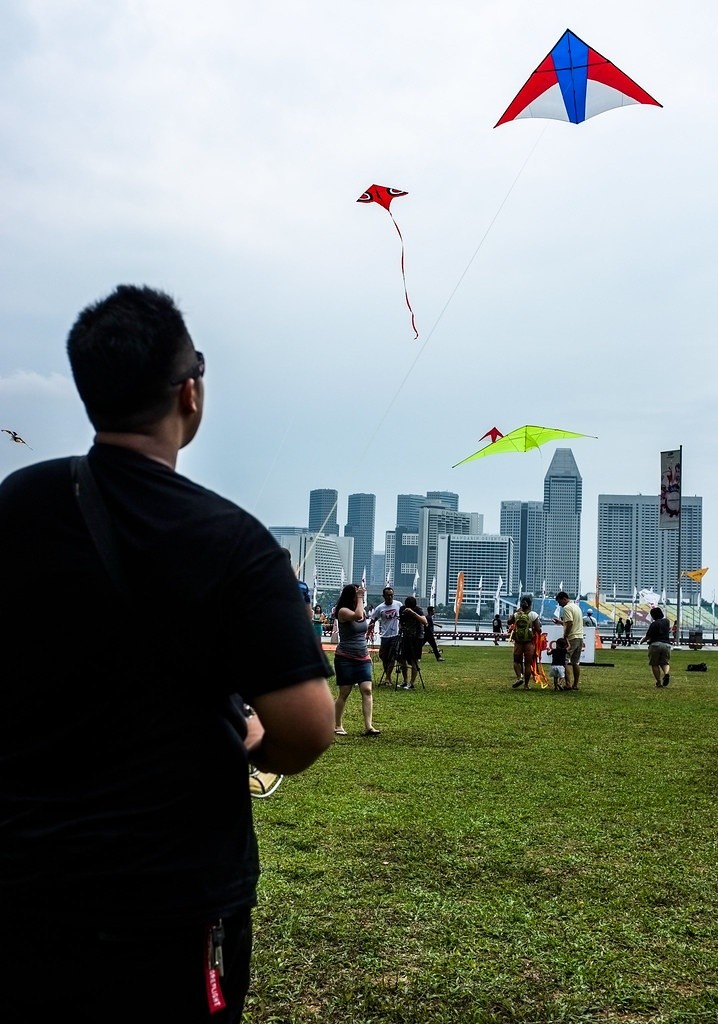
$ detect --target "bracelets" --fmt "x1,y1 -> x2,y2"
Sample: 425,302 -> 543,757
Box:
357,597 -> 362,598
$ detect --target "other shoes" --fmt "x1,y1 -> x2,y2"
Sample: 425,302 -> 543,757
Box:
384,680 -> 414,690
552,684 -> 579,692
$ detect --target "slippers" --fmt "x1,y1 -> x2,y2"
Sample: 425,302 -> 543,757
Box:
335,726 -> 348,735
365,728 -> 381,735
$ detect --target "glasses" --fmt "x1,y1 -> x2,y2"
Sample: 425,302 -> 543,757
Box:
169,351 -> 204,385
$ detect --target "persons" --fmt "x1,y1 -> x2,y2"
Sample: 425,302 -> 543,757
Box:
283,548 -> 339,633
547,592 -> 583,690
667,463 -> 680,486
333,584 -> 381,733
672,620 -> 677,638
583,609 -> 597,627
616,618 -> 631,646
640,608 -> 671,686
493,614 -> 504,645
0,284 -> 335,1024
365,588 -> 446,688
507,595 -> 542,689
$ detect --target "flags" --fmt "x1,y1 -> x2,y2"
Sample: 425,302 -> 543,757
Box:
312,567 -> 716,626
659,450 -> 680,529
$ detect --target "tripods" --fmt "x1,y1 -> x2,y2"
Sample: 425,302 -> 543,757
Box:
377,629 -> 426,691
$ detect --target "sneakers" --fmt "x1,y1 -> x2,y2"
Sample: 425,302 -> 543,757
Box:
656,681 -> 663,688
524,685 -> 530,691
663,673 -> 670,686
512,679 -> 523,688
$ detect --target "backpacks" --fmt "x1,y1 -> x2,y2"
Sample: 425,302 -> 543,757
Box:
515,610 -> 531,641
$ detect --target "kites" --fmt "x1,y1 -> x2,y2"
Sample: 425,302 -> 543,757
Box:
356,184 -> 418,339
493,28 -> 664,129
686,568 -> 709,582
531,633 -> 548,688
1,430 -> 25,444
452,425 -> 598,469
